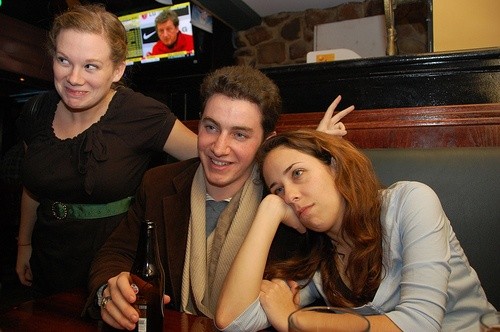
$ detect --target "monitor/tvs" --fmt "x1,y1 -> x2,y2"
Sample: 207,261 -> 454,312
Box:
116,2 -> 196,67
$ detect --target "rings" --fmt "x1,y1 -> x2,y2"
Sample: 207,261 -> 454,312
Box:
99,296 -> 111,309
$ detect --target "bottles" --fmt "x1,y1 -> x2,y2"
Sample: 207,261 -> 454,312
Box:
129,219 -> 165,332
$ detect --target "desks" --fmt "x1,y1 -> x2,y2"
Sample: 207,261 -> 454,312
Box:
0,278 -> 218,332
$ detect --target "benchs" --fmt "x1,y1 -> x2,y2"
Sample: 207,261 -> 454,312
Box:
177,146 -> 500,310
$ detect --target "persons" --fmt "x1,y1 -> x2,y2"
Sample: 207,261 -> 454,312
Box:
89,64 -> 282,331
147,10 -> 195,55
8,6 -> 354,310
214,129 -> 497,330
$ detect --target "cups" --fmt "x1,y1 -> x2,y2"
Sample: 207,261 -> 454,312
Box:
479,312 -> 500,332
288,306 -> 370,332
